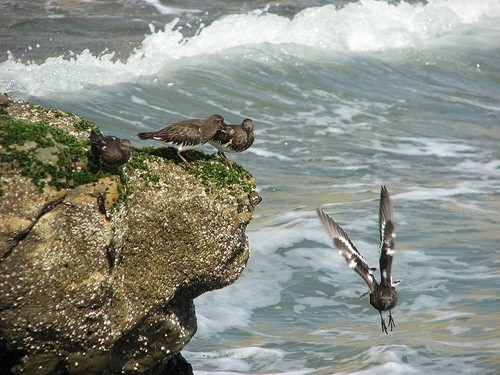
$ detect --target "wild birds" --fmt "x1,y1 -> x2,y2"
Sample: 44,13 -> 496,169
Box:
136,114 -> 228,169
208,119 -> 255,168
90,129 -> 132,177
316,184 -> 399,335
